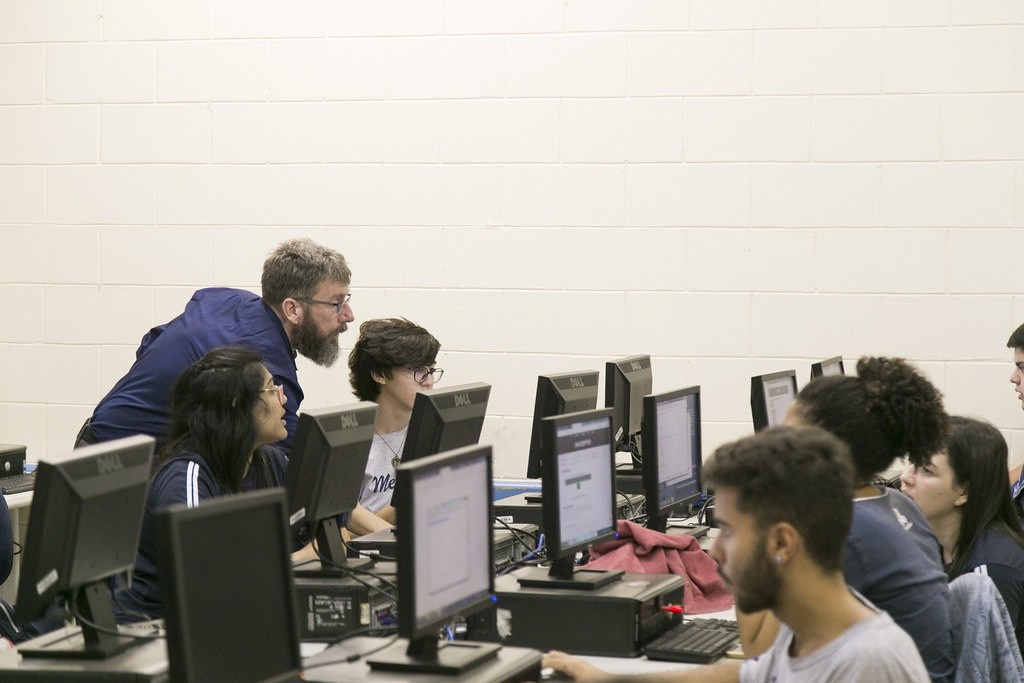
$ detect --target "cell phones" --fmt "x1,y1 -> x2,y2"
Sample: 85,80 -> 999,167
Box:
726,643 -> 746,659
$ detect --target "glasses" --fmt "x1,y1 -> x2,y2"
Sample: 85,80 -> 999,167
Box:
392,364 -> 444,383
291,293 -> 352,315
257,384 -> 284,405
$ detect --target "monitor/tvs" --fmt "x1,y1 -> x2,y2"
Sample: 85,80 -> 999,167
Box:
14,353 -> 847,683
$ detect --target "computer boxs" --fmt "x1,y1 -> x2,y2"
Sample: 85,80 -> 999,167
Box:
0,475 -> 715,683
0,451 -> 25,478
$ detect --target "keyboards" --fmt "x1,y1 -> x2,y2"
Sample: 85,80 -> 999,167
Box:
643,618 -> 741,662
0,473 -> 36,494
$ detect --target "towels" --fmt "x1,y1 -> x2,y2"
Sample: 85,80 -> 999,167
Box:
575,518 -> 734,616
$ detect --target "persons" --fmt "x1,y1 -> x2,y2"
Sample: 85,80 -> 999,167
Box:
341,318 -> 444,542
72,238 -> 354,461
900,411 -> 1024,668
113,347 -> 350,626
735,355 -> 952,683
1008,323 -> 1024,513
542,425 -> 931,683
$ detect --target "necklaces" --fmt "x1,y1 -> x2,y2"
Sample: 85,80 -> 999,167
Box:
375,411 -> 412,469
